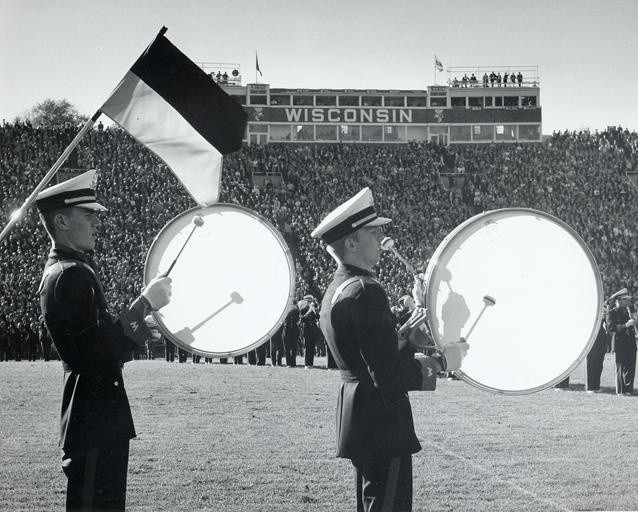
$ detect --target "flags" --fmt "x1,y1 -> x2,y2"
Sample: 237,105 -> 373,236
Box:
256,55 -> 262,77
435,57 -> 444,71
98,33 -> 249,207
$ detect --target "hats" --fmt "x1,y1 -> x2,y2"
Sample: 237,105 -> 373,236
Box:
610,287 -> 631,299
25,169 -> 110,212
310,185 -> 392,248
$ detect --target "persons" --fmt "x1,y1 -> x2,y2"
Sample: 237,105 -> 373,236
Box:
310,186 -> 471,512
216,71 -> 222,85
25,168 -> 170,512
222,73 -> 229,85
451,71 -> 524,87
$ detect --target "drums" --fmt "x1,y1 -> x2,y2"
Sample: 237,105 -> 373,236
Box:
422,207 -> 606,396
142,202 -> 296,360
